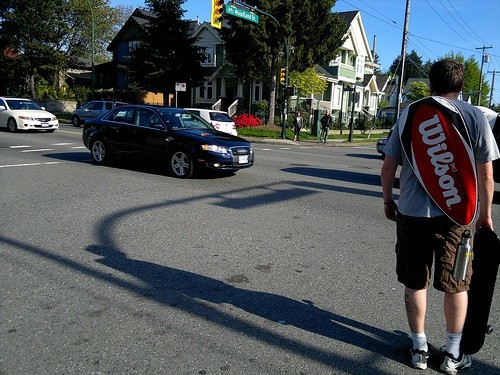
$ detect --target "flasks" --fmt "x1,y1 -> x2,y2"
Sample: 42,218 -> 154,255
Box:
453,230 -> 472,280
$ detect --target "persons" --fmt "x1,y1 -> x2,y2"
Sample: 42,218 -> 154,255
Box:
317,110 -> 332,142
381,61 -> 500,375
292,112 -> 303,141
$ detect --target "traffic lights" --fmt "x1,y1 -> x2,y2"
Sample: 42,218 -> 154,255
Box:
279,68 -> 286,85
210,1 -> 223,30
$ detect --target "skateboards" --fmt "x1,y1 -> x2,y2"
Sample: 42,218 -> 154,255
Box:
461,226 -> 500,356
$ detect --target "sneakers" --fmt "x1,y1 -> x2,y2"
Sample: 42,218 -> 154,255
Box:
439,350 -> 474,373
408,341 -> 436,370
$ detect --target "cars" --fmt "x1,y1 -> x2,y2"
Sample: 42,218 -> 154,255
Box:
82,103 -> 255,179
180,108 -> 238,137
0,96 -> 59,133
71,100 -> 128,128
